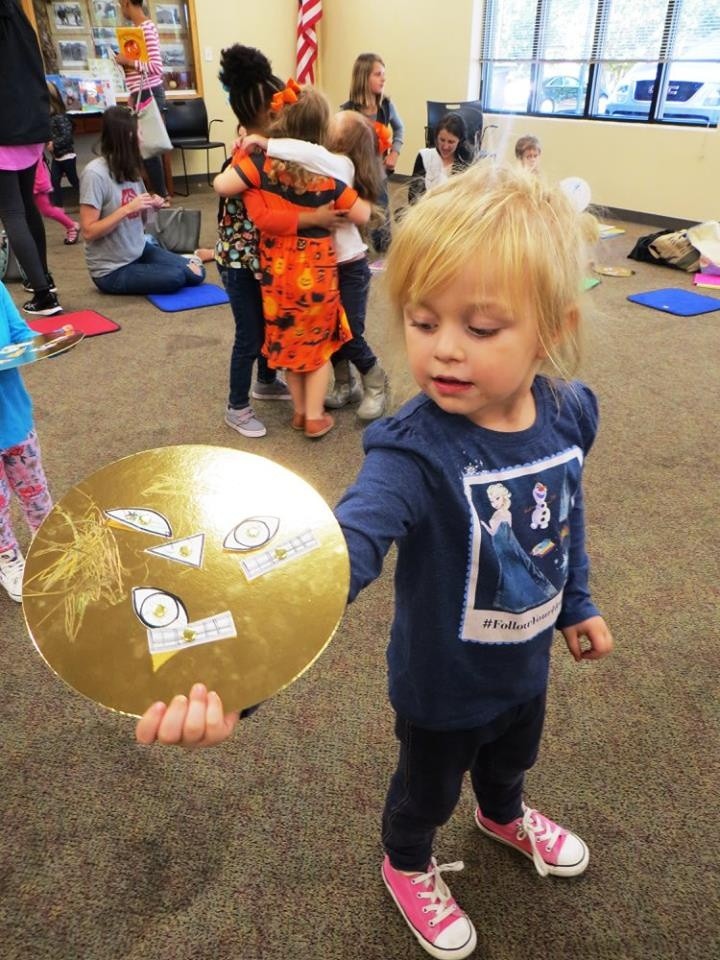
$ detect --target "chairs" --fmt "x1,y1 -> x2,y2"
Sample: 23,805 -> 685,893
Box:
164,97 -> 226,198
424,99 -> 483,148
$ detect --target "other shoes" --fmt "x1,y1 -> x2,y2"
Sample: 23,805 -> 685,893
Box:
64,222 -> 79,243
162,202 -> 170,207
291,413 -> 304,430
304,416 -> 334,437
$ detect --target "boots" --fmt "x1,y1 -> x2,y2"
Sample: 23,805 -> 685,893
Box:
356,360 -> 388,420
325,360 -> 360,407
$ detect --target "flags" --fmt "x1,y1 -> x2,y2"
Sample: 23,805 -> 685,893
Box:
295,0 -> 324,87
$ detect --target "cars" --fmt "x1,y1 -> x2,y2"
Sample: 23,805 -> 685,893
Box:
605,49 -> 720,128
525,75 -> 609,116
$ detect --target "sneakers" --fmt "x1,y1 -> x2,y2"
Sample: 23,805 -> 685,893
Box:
0,549 -> 25,602
20,272 -> 58,292
474,800 -> 590,876
381,851 -> 479,959
22,288 -> 63,315
225,405 -> 266,437
252,377 -> 293,399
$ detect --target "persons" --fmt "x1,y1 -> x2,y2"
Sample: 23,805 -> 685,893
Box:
135,162 -> 612,960
0,280 -> 53,602
0,0 -> 542,439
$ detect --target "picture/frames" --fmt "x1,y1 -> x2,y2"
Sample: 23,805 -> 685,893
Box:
46,0 -> 191,72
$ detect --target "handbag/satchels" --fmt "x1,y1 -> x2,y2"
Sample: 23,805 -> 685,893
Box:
134,67 -> 174,159
648,229 -> 700,274
628,229 -> 682,270
686,219 -> 720,275
157,207 -> 201,254
